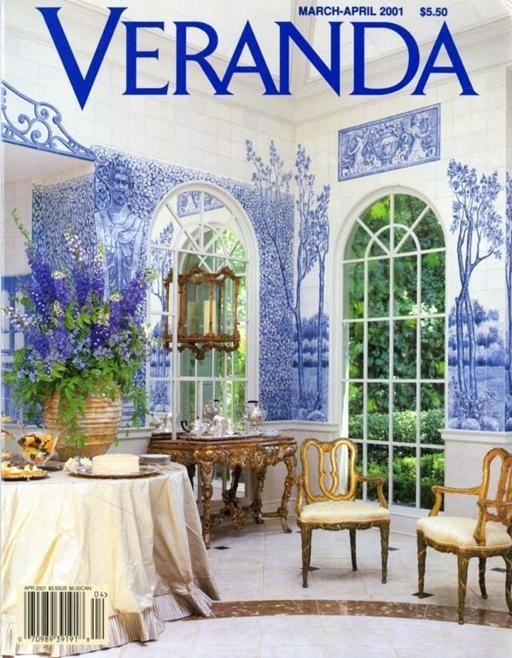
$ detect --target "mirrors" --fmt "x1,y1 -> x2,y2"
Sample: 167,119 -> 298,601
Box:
142,177 -> 263,517
1,78 -> 98,461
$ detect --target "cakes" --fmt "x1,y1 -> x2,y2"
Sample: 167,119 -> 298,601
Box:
91,453 -> 139,475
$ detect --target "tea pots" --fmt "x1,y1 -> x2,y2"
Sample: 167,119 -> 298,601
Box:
182,398 -> 267,438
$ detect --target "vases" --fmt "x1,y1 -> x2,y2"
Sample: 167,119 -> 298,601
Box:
44,375 -> 123,460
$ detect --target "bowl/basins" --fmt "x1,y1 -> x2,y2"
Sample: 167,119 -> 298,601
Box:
139,454 -> 171,465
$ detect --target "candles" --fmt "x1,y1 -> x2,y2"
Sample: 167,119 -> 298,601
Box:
186,299 -> 198,335
202,296 -> 218,336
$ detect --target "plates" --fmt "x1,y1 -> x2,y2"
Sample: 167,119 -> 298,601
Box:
71,465 -> 159,479
2,469 -> 47,482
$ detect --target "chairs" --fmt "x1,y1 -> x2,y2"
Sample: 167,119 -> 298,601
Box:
413,447 -> 511,625
293,437 -> 391,589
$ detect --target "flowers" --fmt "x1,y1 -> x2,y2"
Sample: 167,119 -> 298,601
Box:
1,207 -> 164,452
